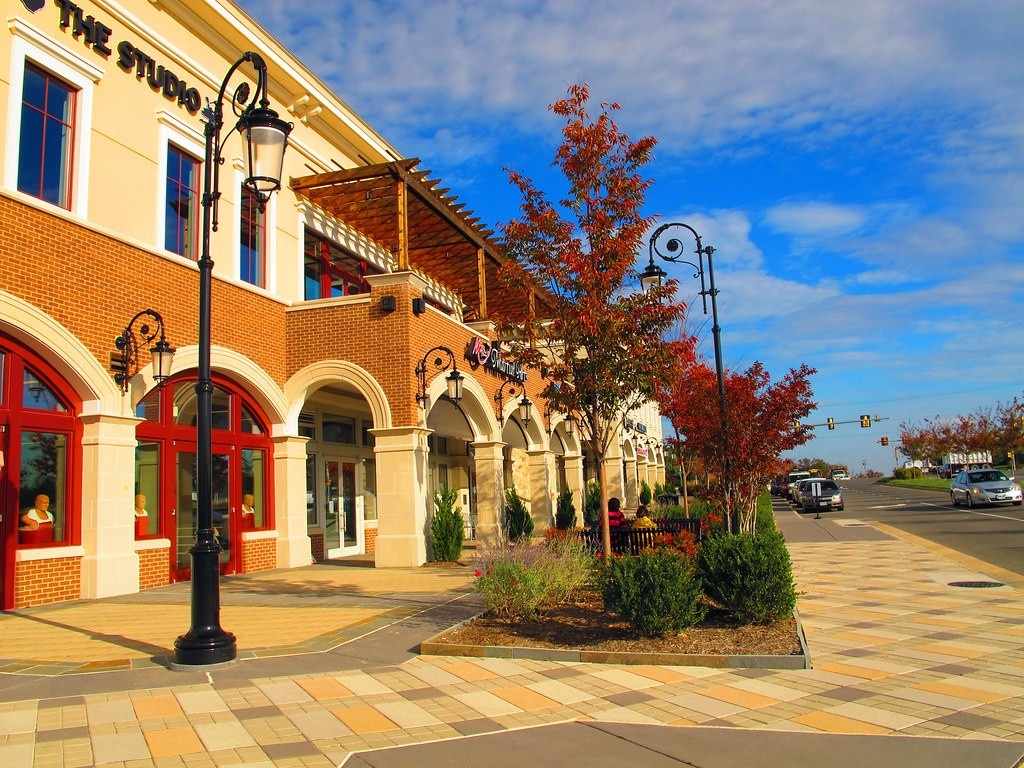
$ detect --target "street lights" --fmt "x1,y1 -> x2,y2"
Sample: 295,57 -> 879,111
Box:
170,49 -> 293,667
636,222 -> 740,536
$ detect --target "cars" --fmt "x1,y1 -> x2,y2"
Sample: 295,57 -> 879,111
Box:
950,468 -> 1023,509
766,468 -> 851,513
939,464 -> 965,479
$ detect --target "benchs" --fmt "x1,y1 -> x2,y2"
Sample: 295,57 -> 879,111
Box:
575,517 -> 699,557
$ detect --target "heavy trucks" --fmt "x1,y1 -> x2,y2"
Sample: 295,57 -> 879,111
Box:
903,448 -> 994,477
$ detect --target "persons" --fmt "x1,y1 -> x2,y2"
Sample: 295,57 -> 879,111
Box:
135,494 -> 147,517
242,494 -> 255,517
598,497 -> 629,548
631,505 -> 658,544
21,494 -> 55,528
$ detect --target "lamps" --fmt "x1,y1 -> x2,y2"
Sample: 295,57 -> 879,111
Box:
632,430 -> 651,445
620,427 -> 629,436
415,347 -> 465,411
110,309 -> 177,394
650,436 -> 660,449
544,405 -> 578,438
495,380 -> 533,428
